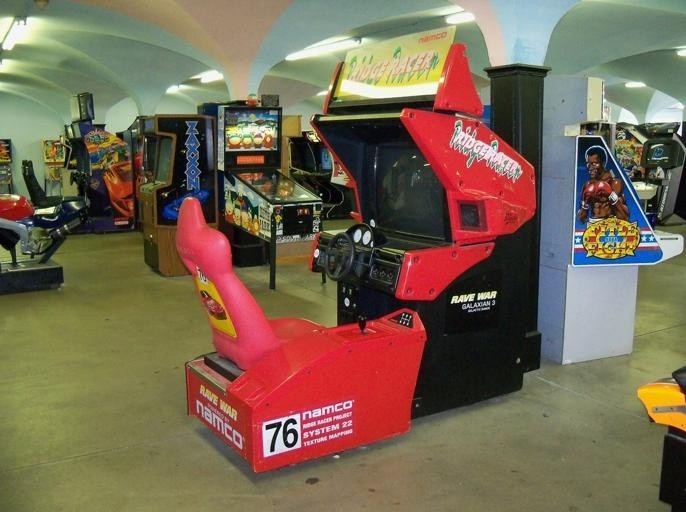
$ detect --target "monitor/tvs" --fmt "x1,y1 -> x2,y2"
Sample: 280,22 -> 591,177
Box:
317,147 -> 332,172
370,139 -> 450,242
640,138 -> 683,168
154,138 -> 173,182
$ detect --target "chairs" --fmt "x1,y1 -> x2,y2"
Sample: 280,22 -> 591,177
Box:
169,195 -> 326,382
19,156 -> 63,209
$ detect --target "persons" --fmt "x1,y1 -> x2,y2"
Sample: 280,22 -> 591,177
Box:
578,146 -> 629,230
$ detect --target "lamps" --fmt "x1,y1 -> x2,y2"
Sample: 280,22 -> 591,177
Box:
281,29 -> 362,63
0,14 -> 29,52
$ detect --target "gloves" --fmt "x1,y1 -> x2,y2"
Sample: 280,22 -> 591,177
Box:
597,179 -> 619,205
580,181 -> 597,211
